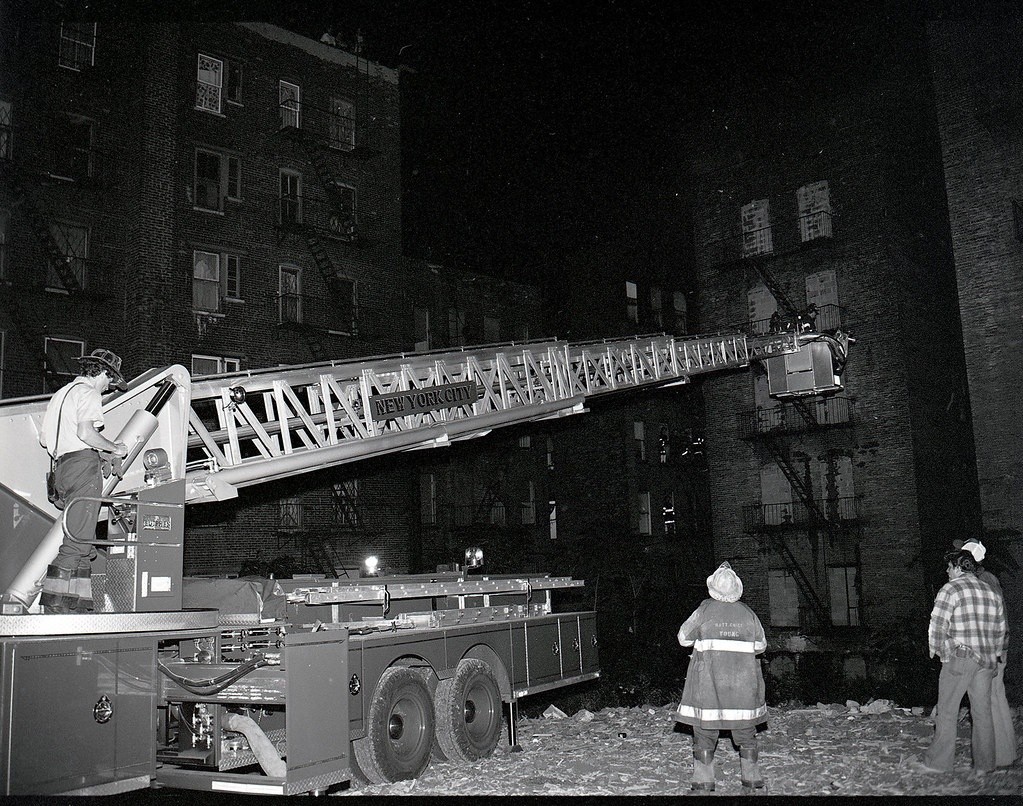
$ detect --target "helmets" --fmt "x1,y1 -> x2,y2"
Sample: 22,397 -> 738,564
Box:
706,567 -> 743,603
70,349 -> 128,391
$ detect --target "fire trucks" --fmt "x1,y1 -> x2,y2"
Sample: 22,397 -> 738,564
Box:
0,322 -> 861,800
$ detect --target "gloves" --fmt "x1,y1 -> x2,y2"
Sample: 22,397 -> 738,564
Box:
102,455 -> 112,478
111,458 -> 124,479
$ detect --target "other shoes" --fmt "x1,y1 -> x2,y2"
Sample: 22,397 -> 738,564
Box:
986,764 -> 1016,775
911,762 -> 945,773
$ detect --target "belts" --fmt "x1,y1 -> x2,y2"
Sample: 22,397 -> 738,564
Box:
56,450 -> 100,463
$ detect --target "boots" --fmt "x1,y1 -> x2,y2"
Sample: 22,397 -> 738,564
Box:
740,748 -> 763,790
687,750 -> 716,796
41,566 -> 95,615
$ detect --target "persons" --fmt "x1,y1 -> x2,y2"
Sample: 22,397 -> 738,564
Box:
320,27 -> 367,56
673,565 -> 770,791
918,537 -> 1018,782
442,326 -> 479,349
769,303 -> 821,336
636,309 -> 690,340
39,348 -> 129,614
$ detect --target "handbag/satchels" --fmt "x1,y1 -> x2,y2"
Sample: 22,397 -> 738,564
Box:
46,472 -> 59,504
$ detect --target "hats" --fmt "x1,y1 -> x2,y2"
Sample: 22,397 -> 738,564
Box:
953,539 -> 986,562
